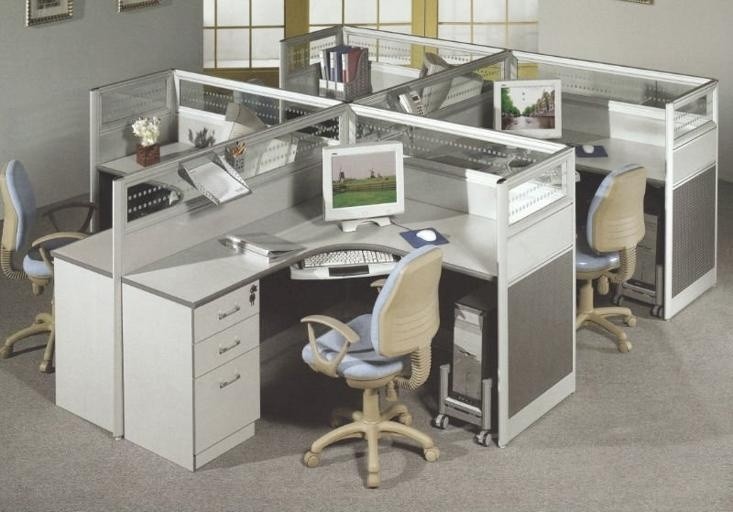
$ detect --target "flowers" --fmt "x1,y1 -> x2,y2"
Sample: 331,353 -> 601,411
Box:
132,115 -> 160,147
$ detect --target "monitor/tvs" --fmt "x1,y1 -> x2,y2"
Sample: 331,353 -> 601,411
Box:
493,79 -> 562,150
322,140 -> 405,233
215,103 -> 299,180
417,52 -> 483,113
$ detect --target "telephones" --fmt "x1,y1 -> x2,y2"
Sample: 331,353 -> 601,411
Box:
398,90 -> 429,117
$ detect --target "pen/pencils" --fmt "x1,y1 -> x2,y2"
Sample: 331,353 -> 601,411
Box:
188,127 -> 216,149
224,140 -> 248,157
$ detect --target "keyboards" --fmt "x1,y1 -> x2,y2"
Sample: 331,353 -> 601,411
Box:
300,249 -> 401,269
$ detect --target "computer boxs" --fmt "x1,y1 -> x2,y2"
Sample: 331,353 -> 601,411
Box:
450,278 -> 497,410
626,187 -> 665,291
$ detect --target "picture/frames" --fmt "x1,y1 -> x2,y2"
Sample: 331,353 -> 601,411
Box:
24,0 -> 76,27
118,0 -> 162,14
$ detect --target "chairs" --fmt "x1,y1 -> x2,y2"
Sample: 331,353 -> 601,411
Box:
0,161 -> 96,373
576,165 -> 646,354
299,245 -> 442,487
232,79 -> 279,129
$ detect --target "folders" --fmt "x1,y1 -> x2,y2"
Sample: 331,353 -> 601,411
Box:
329,47 -> 351,81
225,232 -> 304,258
319,48 -> 330,81
340,47 -> 362,83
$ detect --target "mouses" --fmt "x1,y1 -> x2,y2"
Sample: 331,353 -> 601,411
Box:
416,229 -> 437,242
583,144 -> 594,154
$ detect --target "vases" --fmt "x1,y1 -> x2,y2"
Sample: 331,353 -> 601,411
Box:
137,142 -> 160,166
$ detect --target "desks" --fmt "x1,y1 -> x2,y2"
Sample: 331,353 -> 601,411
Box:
51,70 -> 347,442
343,51 -> 718,321
170,24 -> 509,131
122,103 -> 574,472
